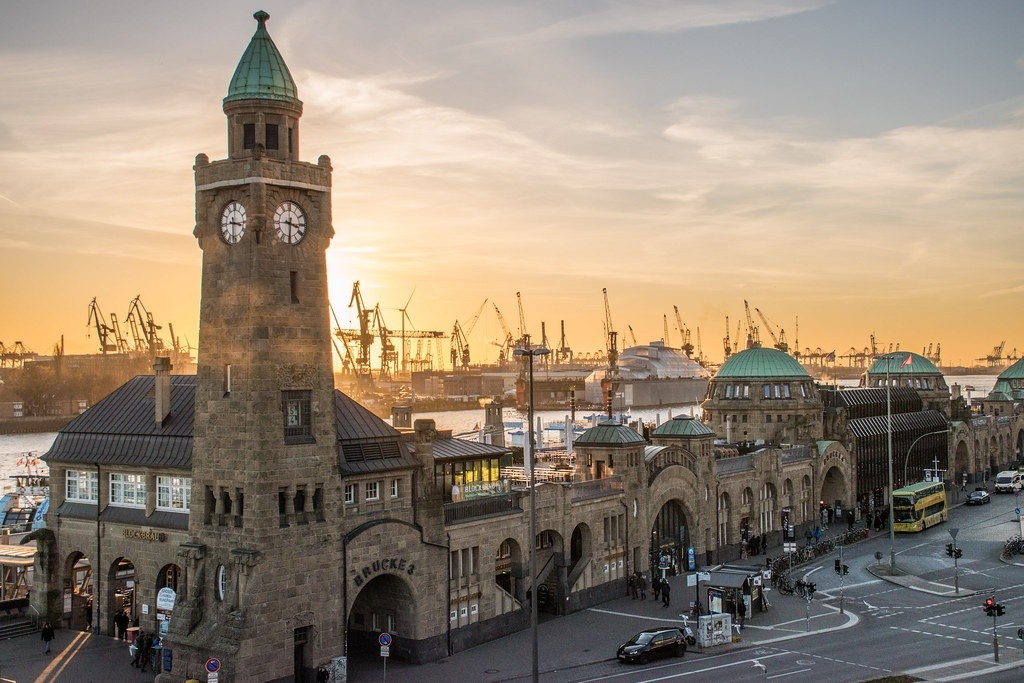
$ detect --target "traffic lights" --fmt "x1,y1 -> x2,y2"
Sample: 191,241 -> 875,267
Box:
983,599 -> 1005,616
843,564 -> 849,575
946,543 -> 953,558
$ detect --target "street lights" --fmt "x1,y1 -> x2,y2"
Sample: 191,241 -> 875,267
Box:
513,347 -> 550,683
905,430 -> 949,486
881,356 -> 896,576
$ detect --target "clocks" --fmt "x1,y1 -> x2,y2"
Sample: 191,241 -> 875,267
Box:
220,200 -> 247,246
273,200 -> 308,245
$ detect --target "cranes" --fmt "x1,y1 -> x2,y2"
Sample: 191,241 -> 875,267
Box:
722,298 -> 801,361
329,280 -> 488,378
600,288 -> 704,368
492,290 -> 573,366
86,294 -> 164,354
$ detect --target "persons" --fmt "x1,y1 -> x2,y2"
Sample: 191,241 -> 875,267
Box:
761,533 -> 767,555
874,502 -> 889,532
847,511 -> 855,531
661,578 -> 670,608
814,525 -> 821,542
960,469 -> 968,492
748,535 -> 760,556
115,608 -> 129,642
724,591 -> 746,630
41,623 -> 55,654
81,599 -> 92,629
628,572 -> 638,600
130,630 -> 159,673
804,527 -> 813,542
652,574 -> 662,600
636,572 -> 646,600
866,511 -> 873,530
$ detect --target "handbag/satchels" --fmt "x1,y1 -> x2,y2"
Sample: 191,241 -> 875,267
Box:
650,588 -> 655,595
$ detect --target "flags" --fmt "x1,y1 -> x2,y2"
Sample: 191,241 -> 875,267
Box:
900,353 -> 912,369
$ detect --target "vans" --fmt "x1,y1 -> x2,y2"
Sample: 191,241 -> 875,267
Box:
994,467 -> 1024,493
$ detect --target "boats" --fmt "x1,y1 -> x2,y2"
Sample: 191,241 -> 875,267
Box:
964,385 -> 976,391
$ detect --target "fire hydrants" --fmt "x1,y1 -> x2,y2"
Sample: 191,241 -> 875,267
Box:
865,529 -> 869,538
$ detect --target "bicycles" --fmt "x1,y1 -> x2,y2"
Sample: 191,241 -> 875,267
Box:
1003,534 -> 1021,558
771,529 -> 865,601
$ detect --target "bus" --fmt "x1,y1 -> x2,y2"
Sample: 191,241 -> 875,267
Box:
893,481 -> 948,532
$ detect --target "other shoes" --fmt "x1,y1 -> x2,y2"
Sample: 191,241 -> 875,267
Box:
45,650 -> 50,654
655,596 -> 658,600
136,665 -> 141,668
141,669 -> 147,672
130,663 -> 134,666
663,604 -> 669,607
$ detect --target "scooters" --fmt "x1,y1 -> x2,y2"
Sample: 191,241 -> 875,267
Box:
673,614 -> 696,645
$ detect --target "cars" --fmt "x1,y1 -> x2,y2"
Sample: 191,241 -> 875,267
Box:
617,627 -> 688,664
966,491 -> 990,505
